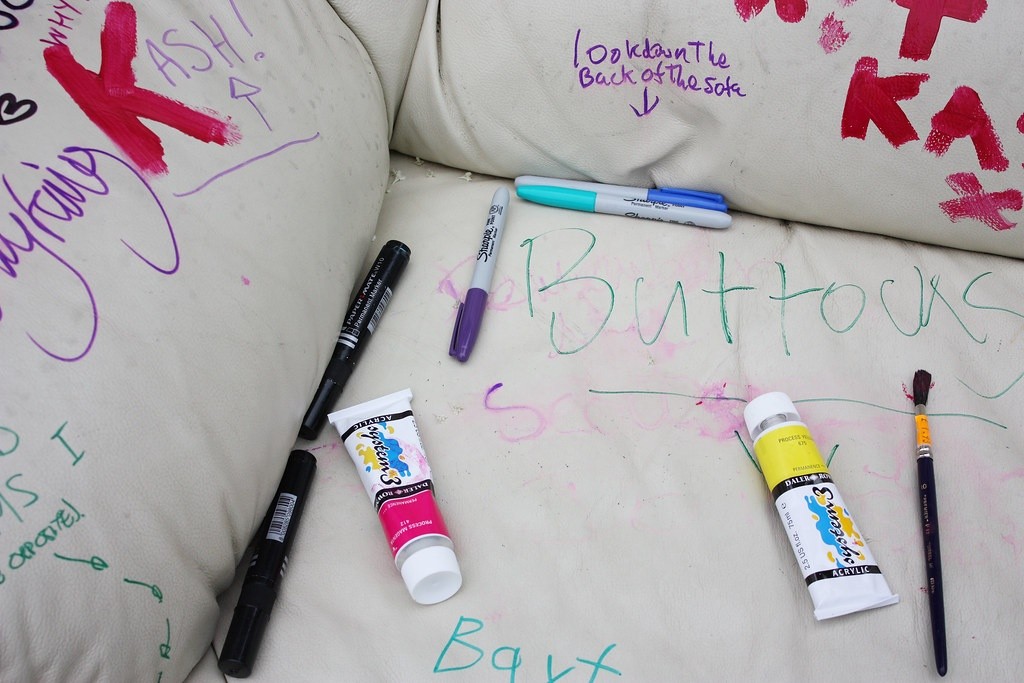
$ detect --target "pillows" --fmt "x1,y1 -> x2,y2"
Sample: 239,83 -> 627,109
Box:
387,0 -> 1024,260
0,0 -> 391,683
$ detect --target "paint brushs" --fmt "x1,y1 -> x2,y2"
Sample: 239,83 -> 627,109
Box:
912,368 -> 949,677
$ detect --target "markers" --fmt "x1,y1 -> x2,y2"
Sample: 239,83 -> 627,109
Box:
216,448 -> 316,678
295,239 -> 410,440
446,187 -> 512,361
515,173 -> 734,230
